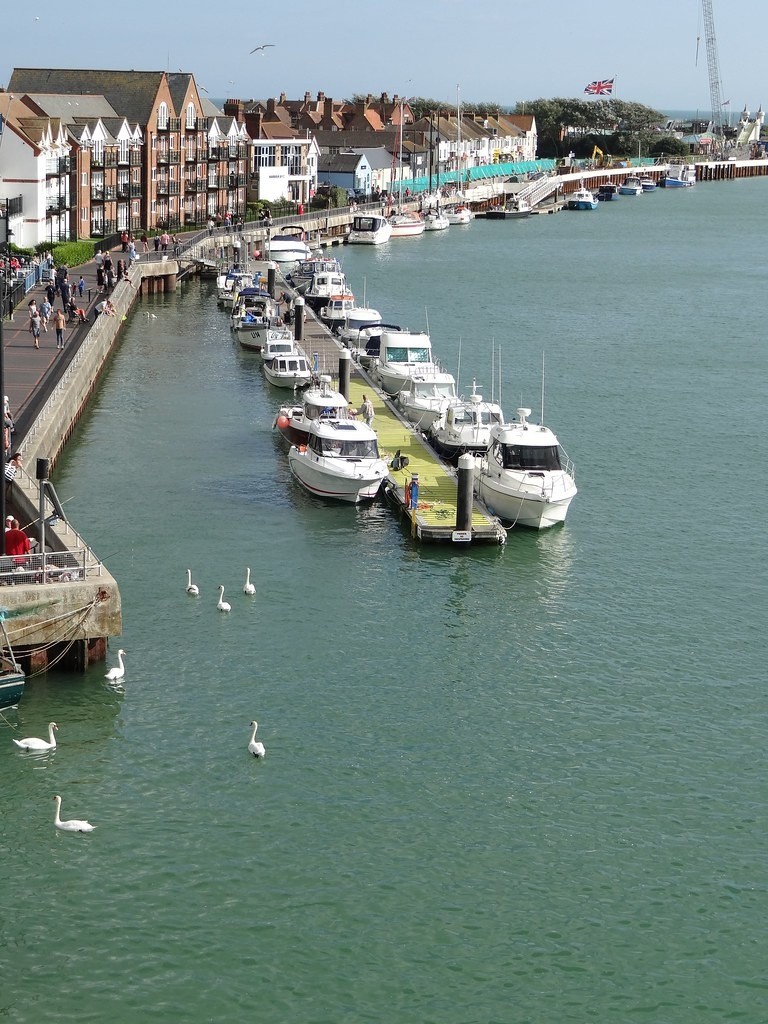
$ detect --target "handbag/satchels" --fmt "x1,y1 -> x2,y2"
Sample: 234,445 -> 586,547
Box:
4,460 -> 16,482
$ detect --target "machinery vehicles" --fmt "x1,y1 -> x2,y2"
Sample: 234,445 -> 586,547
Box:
694,0 -> 726,155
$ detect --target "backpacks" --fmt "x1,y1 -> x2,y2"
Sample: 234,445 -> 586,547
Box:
48,286 -> 53,294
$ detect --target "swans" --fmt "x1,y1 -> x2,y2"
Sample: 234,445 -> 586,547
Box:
216,585 -> 232,612
242,567 -> 257,596
13,722 -> 59,753
104,649 -> 127,680
146,311 -> 157,319
185,568 -> 199,596
51,795 -> 98,835
247,720 -> 265,759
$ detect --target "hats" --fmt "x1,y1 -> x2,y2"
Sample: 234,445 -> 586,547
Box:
5,515 -> 14,522
63,279 -> 67,282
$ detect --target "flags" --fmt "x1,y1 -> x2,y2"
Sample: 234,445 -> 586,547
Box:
584,78 -> 615,95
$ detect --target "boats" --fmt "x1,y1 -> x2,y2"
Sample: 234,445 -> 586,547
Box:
215,82 -> 698,530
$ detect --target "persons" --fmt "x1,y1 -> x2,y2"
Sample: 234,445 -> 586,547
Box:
229,171 -> 236,186
276,291 -> 293,310
5,453 -> 22,495
205,211 -> 245,236
352,200 -> 357,211
371,184 -> 455,207
259,206 -> 274,228
4,395 -> 17,435
4,515 -> 32,569
0,250 -> 128,350
490,203 -> 501,210
353,395 -> 375,426
122,231 -> 182,265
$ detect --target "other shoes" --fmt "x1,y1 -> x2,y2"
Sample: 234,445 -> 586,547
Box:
61,345 -> 64,349
35,343 -> 40,349
6,500 -> 9,504
12,432 -> 16,435
57,345 -> 60,349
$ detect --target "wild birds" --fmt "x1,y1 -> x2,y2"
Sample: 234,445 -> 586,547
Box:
197,83 -> 208,94
249,44 -> 276,54
228,81 -> 235,87
